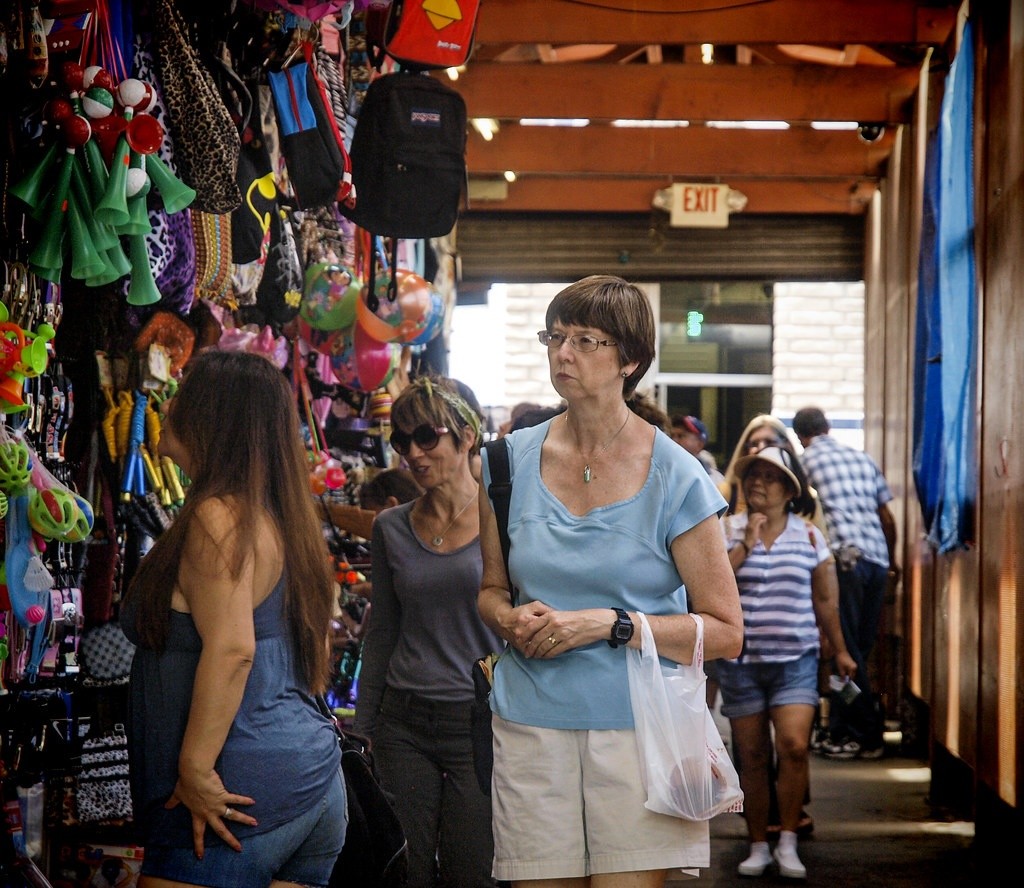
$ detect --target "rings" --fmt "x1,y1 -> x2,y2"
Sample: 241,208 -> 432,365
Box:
224,808 -> 232,818
548,637 -> 557,646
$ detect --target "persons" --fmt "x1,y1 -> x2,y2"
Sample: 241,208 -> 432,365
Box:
719,447 -> 857,877
119,352 -> 349,888
477,276 -> 744,888
793,407 -> 901,760
315,468 -> 427,541
352,377 -> 508,888
720,414 -> 839,840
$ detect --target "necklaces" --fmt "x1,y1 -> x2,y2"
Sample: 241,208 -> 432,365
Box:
423,491 -> 479,546
566,408 -> 629,483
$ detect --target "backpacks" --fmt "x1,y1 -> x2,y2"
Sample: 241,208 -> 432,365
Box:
338,61 -> 466,310
380,0 -> 481,73
266,58 -> 352,210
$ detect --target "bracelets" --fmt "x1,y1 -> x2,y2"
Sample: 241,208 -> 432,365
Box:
741,541 -> 749,557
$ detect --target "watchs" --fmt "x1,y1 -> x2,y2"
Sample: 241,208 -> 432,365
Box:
607,607 -> 634,649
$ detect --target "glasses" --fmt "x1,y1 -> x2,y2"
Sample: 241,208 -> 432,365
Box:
389,424 -> 449,456
537,330 -> 618,353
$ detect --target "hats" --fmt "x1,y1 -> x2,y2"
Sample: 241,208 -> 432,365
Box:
733,447 -> 802,499
671,414 -> 707,443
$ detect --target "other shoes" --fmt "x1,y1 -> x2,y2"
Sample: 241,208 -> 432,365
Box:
737,850 -> 773,875
774,848 -> 807,877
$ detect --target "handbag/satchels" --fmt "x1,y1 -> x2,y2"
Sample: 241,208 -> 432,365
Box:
468,656 -> 497,797
338,737 -> 406,888
625,611 -> 744,823
